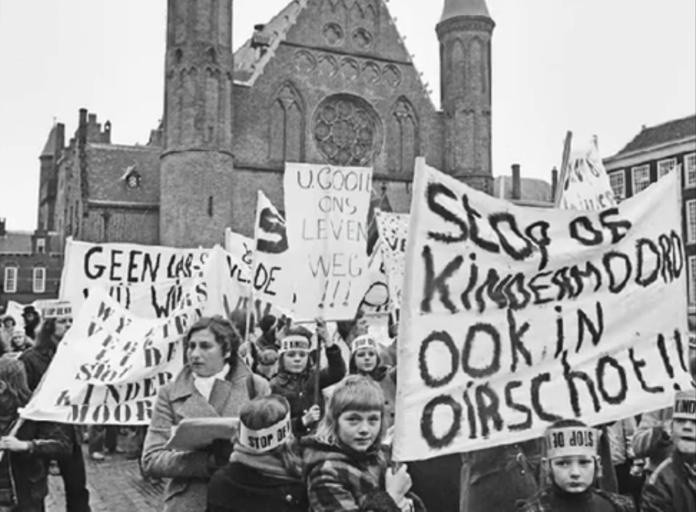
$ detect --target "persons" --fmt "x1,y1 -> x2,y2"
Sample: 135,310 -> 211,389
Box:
140,313 -> 428,512
515,304 -> 696,512
0,300 -> 129,511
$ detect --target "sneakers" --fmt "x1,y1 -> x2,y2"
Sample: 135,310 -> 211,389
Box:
89,451 -> 104,460
109,444 -> 125,453
82,432 -> 90,443
48,463 -> 60,475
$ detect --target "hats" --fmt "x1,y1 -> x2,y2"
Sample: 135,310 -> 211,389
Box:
21,306 -> 39,315
